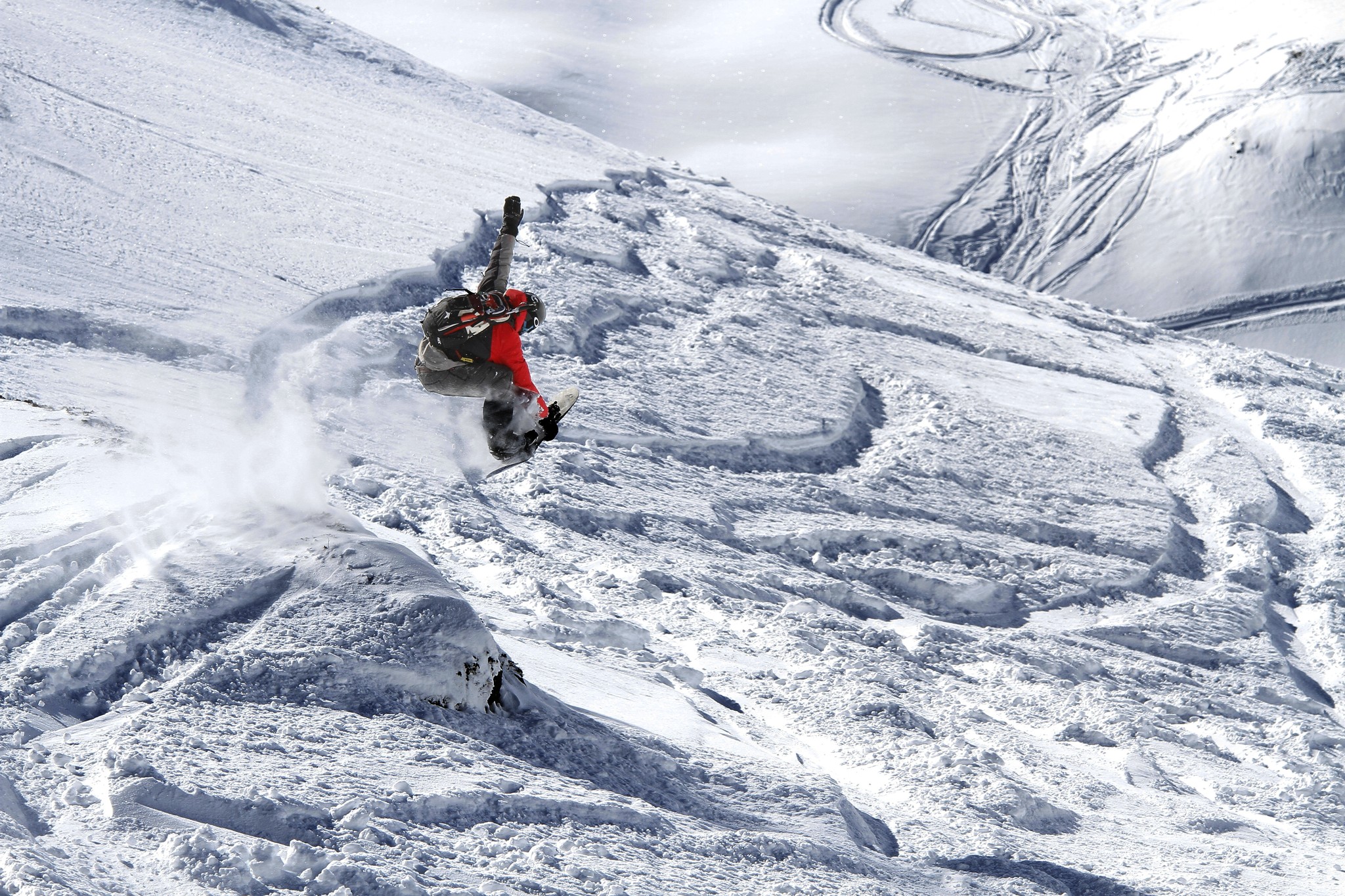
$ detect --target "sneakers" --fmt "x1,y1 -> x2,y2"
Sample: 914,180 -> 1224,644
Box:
489,429 -> 538,461
483,399 -> 513,428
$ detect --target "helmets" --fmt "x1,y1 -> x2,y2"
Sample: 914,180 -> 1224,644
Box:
518,292 -> 546,335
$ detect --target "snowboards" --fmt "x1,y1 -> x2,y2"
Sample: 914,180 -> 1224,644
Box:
485,387 -> 579,479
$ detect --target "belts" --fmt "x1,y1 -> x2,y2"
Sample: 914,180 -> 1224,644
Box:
414,363 -> 433,373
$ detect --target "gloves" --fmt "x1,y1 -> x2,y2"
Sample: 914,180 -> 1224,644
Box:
540,417 -> 559,441
500,196 -> 524,237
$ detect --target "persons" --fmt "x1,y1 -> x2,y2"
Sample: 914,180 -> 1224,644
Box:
414,196 -> 559,460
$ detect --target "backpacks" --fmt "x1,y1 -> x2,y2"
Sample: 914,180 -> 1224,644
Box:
422,290 -> 516,361
